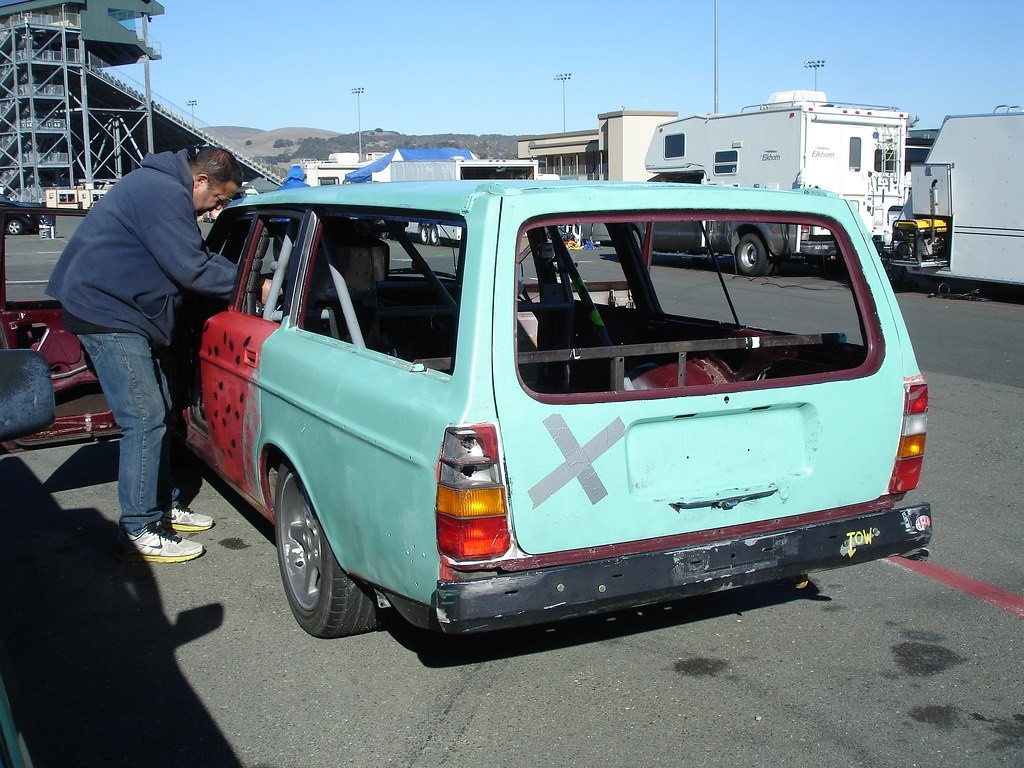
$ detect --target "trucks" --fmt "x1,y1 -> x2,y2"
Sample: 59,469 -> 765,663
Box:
372,160 -> 538,247
633,98 -> 908,274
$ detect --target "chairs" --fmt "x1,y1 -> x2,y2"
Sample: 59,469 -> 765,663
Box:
633,326 -> 797,388
0,348 -> 56,442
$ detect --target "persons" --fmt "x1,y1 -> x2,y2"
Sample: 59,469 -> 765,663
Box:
44,146 -> 283,562
270,165 -> 311,272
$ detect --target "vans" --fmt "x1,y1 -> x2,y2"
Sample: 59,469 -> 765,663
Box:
0,179 -> 935,641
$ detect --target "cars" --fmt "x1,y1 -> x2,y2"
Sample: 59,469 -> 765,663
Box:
0,201 -> 57,236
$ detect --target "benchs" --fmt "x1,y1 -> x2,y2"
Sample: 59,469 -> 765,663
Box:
0,299 -> 126,454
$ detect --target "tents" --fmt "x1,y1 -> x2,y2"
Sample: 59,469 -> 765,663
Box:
344,148 -> 477,183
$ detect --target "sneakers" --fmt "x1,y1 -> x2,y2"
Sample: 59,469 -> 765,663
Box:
119,521 -> 204,562
157,504 -> 215,532
